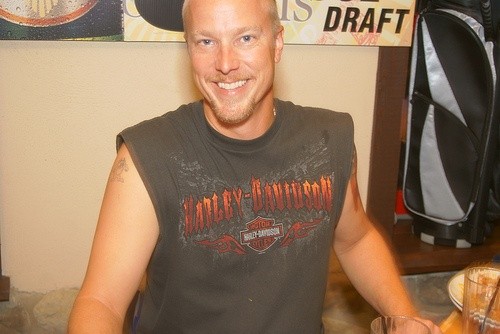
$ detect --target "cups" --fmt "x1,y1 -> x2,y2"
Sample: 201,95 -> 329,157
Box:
460,261 -> 500,334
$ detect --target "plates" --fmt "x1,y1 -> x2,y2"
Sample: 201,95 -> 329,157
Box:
447,266 -> 500,330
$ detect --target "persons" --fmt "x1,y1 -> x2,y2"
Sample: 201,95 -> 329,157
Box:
66,0 -> 442,334
